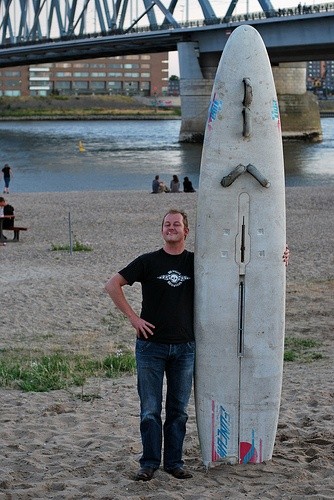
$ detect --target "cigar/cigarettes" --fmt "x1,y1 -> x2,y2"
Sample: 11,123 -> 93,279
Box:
169,232 -> 171,234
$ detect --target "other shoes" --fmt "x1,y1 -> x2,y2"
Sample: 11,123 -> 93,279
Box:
169,467 -> 193,480
6,191 -> 10,194
3,190 -> 5,194
135,465 -> 158,481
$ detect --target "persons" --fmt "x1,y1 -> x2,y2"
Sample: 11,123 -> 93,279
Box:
0,196 -> 16,240
152,175 -> 195,193
104,210 -> 290,480
0,164 -> 14,194
278,2 -> 309,16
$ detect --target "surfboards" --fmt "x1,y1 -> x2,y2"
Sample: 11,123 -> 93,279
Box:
193,23 -> 286,466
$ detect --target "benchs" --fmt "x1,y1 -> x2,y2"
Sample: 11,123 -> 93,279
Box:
3,228 -> 28,242
